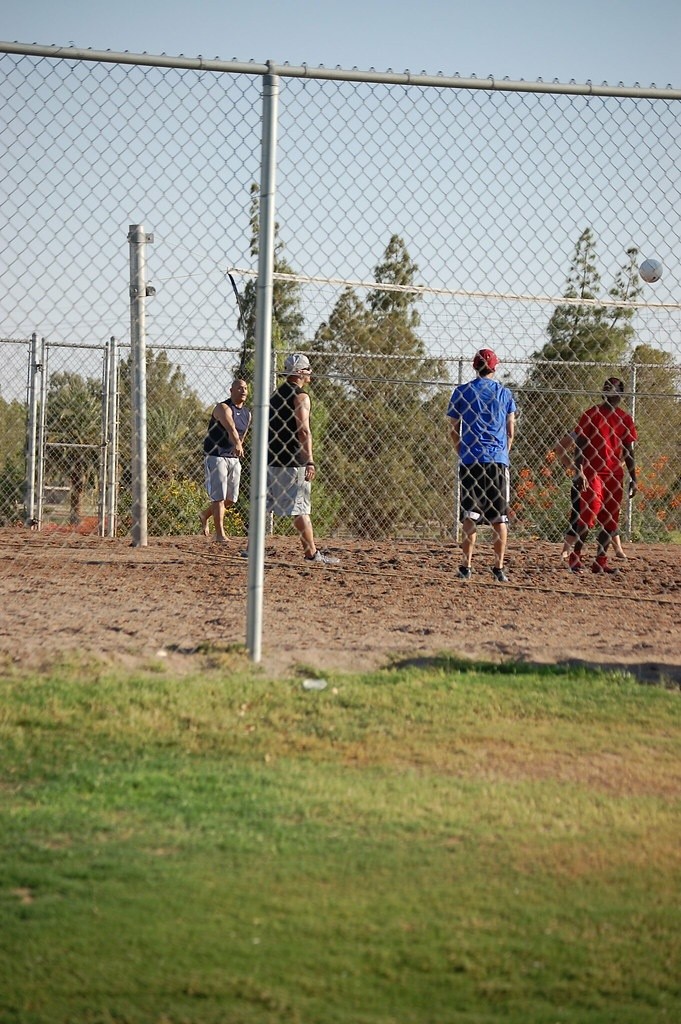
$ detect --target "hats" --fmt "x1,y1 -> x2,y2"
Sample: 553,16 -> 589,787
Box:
474,349 -> 500,370
276,353 -> 310,377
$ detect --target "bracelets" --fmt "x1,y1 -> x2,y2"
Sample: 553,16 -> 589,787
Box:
306,462 -> 316,470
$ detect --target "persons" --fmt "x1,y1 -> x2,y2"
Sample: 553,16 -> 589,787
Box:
199,379 -> 252,542
447,349 -> 518,583
554,377 -> 639,574
240,353 -> 341,565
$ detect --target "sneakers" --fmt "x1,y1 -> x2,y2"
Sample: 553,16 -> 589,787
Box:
241,550 -> 250,557
454,566 -> 472,579
306,550 -> 339,563
492,567 -> 508,581
593,555 -> 615,574
569,550 -> 581,572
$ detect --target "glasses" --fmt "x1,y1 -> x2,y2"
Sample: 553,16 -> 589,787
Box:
302,366 -> 311,371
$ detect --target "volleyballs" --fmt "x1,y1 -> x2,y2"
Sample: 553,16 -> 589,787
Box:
639,259 -> 663,283
